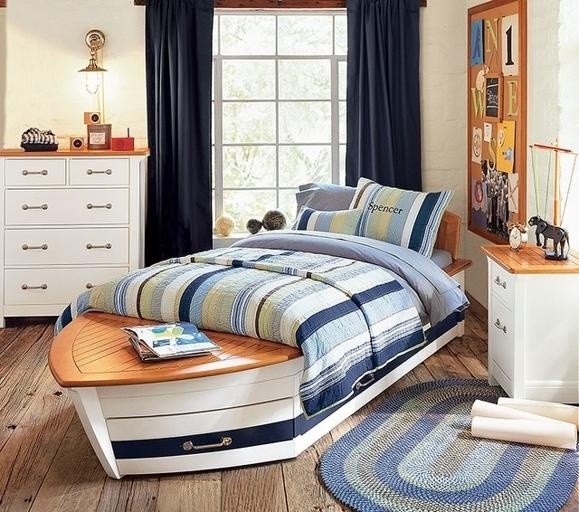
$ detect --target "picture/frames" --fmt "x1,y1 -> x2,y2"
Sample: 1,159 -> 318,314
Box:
482,73 -> 504,124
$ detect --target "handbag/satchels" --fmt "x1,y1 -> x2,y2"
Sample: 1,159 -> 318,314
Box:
19,126 -> 58,152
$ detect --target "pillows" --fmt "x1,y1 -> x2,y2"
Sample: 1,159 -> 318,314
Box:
294,179 -> 354,214
286,204 -> 365,238
344,175 -> 453,266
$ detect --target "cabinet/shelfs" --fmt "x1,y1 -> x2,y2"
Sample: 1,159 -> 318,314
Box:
1,147 -> 151,329
479,242 -> 579,404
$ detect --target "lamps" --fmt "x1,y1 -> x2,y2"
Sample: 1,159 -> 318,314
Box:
72,27 -> 106,93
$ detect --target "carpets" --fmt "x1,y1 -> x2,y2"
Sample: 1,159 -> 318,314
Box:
316,376 -> 577,512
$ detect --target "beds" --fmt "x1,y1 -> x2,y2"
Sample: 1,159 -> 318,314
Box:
49,176 -> 474,482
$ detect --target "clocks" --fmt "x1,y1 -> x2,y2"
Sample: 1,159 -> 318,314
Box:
507,225 -> 530,252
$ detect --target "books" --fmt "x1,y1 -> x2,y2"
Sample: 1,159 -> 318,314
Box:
120,320 -> 222,362
